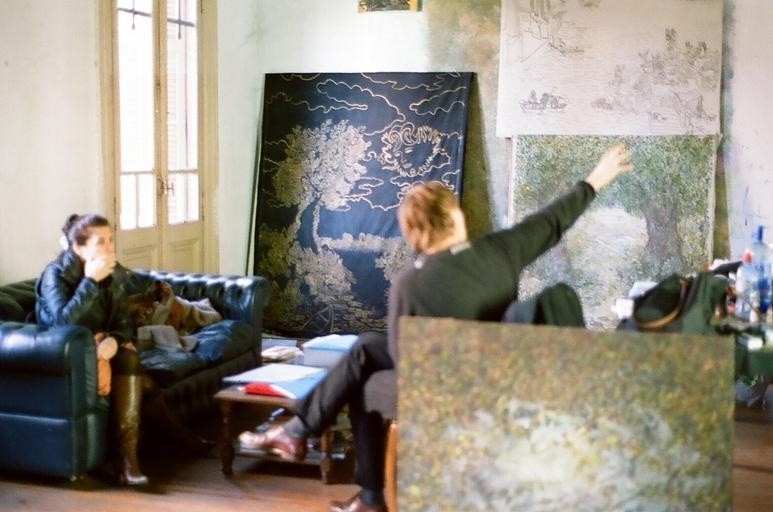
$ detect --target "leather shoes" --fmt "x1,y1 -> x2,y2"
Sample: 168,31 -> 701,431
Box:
238,425 -> 307,465
328,492 -> 387,512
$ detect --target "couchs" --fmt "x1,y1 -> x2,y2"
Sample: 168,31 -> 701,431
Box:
0,267 -> 271,486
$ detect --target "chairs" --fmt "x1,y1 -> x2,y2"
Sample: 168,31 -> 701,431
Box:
382,316 -> 737,511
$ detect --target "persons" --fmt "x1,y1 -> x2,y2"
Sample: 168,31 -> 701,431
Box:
34,214 -> 214,485
237,144 -> 634,512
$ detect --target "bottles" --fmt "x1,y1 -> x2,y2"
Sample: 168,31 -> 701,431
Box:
736,251 -> 759,322
744,223 -> 772,312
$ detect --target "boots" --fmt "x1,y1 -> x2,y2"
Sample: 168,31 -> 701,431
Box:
112,373 -> 151,487
145,389 -> 217,455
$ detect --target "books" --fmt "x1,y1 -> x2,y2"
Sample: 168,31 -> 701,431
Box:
223,330 -> 357,403
